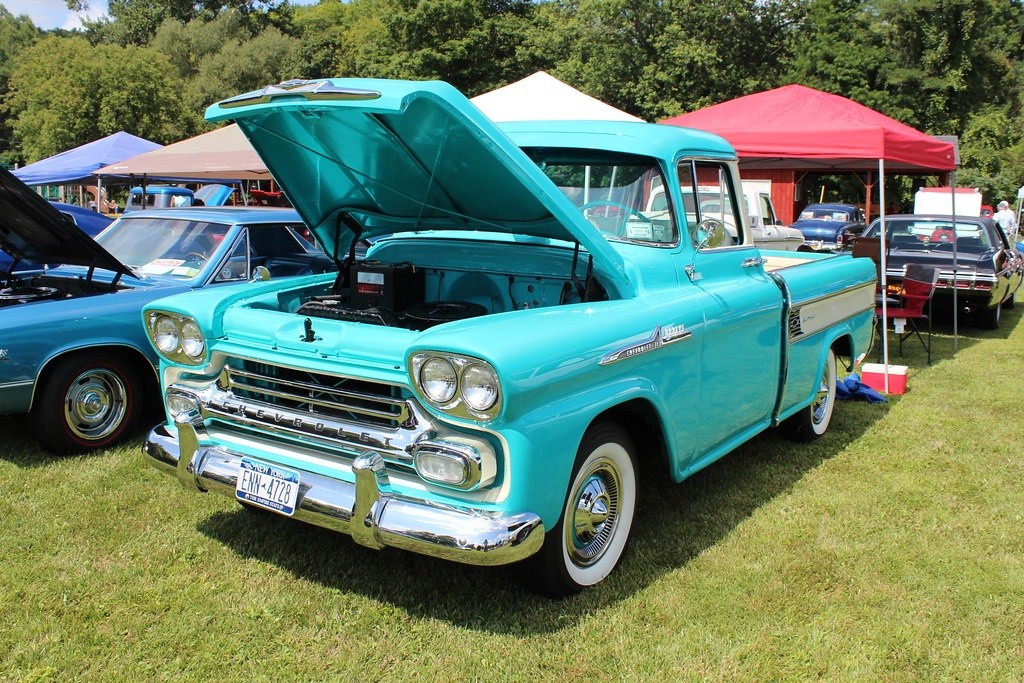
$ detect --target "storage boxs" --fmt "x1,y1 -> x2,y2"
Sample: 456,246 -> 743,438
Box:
802,211 -> 814,219
833,212 -> 847,222
861,362 -> 909,395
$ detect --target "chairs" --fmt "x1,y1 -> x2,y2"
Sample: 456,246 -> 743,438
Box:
875,263 -> 940,365
894,234 -> 919,242
957,237 -> 983,246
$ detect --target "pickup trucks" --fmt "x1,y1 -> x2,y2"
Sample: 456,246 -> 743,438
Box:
143,75 -> 877,598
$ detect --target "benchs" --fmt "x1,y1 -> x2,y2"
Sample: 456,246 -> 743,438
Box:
263,252 -> 364,277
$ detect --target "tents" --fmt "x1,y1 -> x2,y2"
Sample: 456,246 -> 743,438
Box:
9,131 -> 165,214
455,71 -> 647,222
92,109 -> 275,214
655,85 -> 957,396
1014,186 -> 1024,249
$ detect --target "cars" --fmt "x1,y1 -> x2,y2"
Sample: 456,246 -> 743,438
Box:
908,188 -> 993,244
0,163 -> 366,454
790,203 -> 868,254
854,215 -> 1024,331
0,199 -> 169,276
626,184 -> 806,251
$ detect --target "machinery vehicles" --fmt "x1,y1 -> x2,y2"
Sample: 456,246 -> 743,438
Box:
124,183 -> 237,220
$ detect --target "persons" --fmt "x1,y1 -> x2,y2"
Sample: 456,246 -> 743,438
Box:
993,201 -> 1017,246
